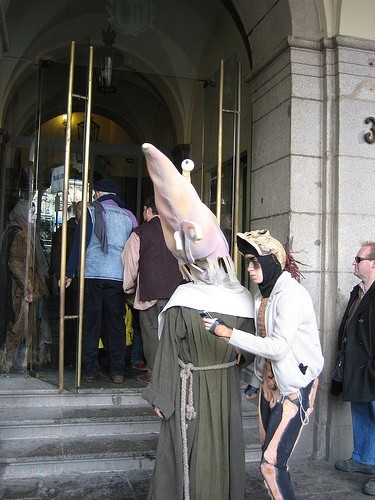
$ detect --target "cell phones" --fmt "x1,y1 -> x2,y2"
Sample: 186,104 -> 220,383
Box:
199,310 -> 213,320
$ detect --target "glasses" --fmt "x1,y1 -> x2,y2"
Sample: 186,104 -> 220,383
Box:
353,256 -> 375,265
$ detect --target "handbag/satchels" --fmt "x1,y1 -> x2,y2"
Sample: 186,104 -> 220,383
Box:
330,350 -> 345,395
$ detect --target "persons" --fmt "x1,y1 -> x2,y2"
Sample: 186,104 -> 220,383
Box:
1,142 -> 375,500
330,240 -> 375,500
198,227 -> 321,500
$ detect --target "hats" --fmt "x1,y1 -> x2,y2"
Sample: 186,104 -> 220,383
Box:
96,180 -> 119,194
237,231 -> 287,273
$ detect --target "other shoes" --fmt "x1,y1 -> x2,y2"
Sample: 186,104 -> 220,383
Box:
85,375 -> 97,382
111,375 -> 123,383
364,475 -> 375,495
335,458 -> 375,474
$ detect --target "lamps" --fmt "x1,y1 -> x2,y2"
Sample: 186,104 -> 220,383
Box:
75,114 -> 103,165
90,0 -> 128,93
